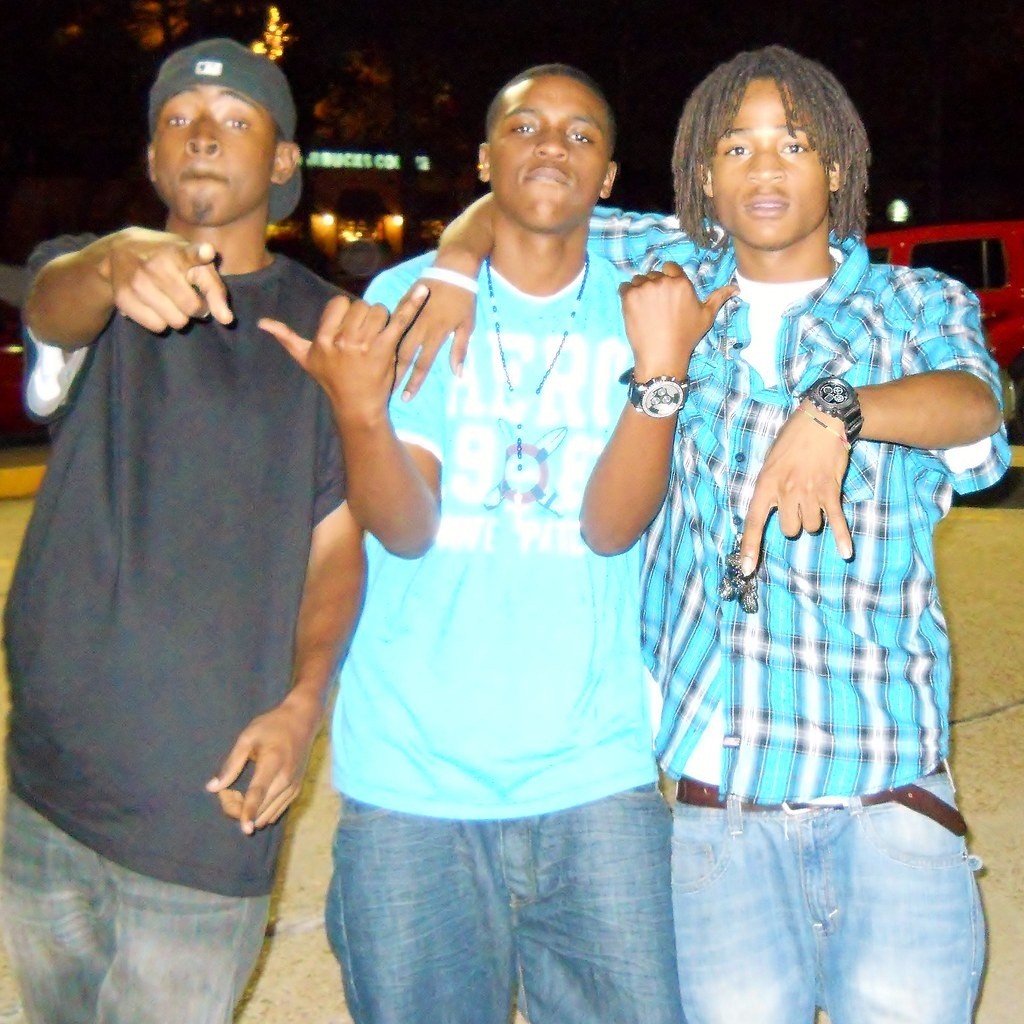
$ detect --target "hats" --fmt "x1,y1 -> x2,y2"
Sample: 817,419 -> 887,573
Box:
146,39 -> 302,220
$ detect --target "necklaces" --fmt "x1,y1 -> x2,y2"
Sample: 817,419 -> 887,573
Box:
721,295 -> 825,614
484,253 -> 590,470
796,405 -> 852,452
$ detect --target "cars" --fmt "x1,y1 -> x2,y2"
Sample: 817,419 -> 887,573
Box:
872,221 -> 1024,374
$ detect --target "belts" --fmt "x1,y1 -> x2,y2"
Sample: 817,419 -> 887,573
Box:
675,761 -> 970,836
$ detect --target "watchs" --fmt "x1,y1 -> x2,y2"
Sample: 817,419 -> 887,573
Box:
619,366 -> 692,418
798,376 -> 865,446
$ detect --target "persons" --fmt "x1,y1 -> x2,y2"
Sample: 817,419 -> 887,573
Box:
380,48 -> 1015,1024
0,36 -> 369,1023
260,57 -> 743,1024
